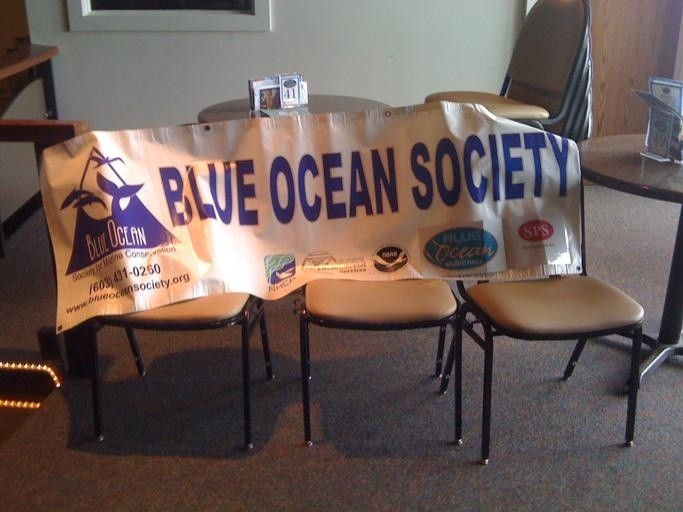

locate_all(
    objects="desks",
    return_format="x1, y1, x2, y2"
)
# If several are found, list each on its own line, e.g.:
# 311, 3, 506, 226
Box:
574, 132, 683, 390
198, 92, 389, 129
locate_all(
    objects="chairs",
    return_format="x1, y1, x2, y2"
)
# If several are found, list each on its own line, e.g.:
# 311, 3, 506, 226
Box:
423, 2, 598, 264
439, 270, 643, 466
87, 294, 276, 451
295, 281, 462, 449
